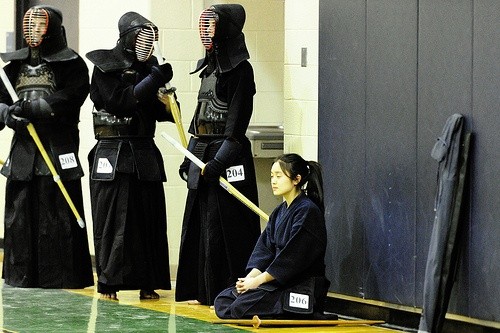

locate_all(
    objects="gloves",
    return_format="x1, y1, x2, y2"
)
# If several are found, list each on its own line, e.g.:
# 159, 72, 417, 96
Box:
180, 161, 190, 181
6, 99, 41, 130
151, 64, 173, 83
202, 160, 223, 181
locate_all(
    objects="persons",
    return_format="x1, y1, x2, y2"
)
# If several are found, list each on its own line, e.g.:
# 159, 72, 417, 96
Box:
85, 12, 182, 300
176, 4, 261, 310
214, 153, 339, 327
0, 5, 94, 289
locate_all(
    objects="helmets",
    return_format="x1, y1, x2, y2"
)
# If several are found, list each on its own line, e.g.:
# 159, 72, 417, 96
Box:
22, 4, 63, 48
119, 12, 158, 63
200, 4, 245, 51
